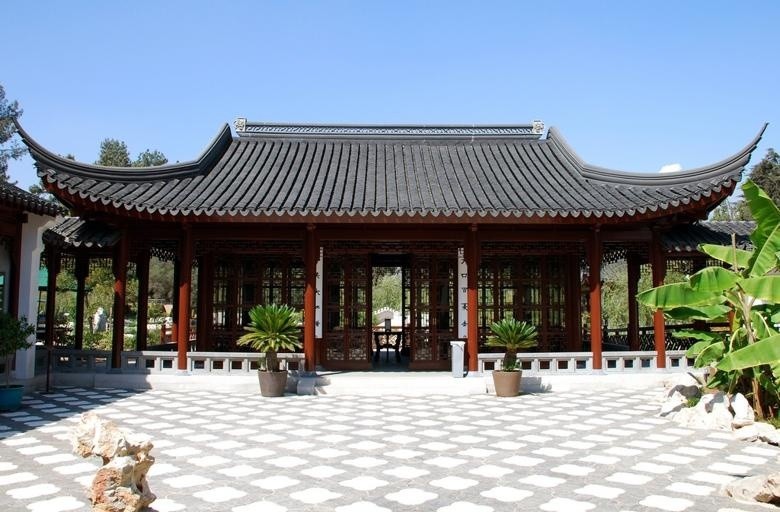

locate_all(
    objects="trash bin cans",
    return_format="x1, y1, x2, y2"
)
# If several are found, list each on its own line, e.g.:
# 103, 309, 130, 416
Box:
450, 340, 466, 378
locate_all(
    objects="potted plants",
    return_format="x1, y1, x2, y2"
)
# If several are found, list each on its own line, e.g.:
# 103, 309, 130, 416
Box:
0, 307, 35, 411
236, 303, 303, 396
484, 318, 539, 396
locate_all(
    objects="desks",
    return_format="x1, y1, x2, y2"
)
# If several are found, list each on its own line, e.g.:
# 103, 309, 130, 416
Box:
373, 332, 402, 360
